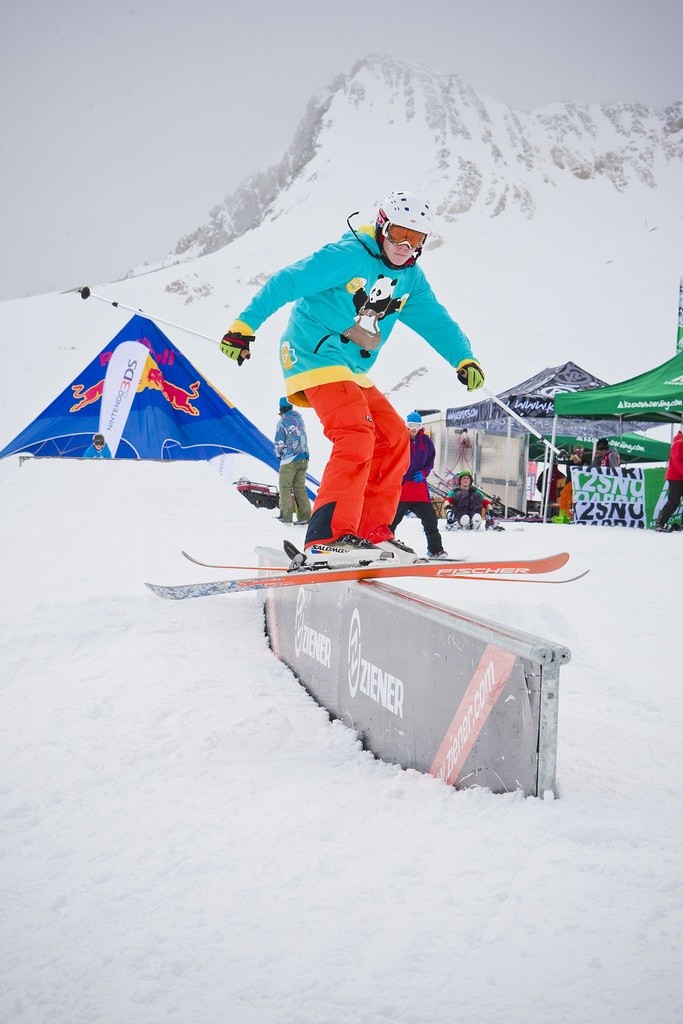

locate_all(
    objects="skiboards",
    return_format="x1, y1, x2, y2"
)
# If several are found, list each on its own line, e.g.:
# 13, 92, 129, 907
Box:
420, 557, 464, 562
147, 548, 592, 601
427, 468, 518, 518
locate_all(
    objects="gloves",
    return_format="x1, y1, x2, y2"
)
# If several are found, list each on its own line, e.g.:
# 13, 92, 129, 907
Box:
412, 468, 427, 483
219, 331, 249, 366
458, 364, 484, 391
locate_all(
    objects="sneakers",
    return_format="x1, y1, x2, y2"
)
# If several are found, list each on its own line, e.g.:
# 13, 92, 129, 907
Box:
372, 538, 417, 565
428, 551, 446, 558
305, 534, 399, 567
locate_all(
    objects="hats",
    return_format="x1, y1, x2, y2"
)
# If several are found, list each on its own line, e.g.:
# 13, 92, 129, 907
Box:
596, 438, 608, 446
406, 412, 422, 422
93, 435, 103, 441
279, 397, 291, 407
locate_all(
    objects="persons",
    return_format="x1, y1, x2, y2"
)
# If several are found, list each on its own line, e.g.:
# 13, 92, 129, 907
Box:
566, 444, 590, 485
588, 438, 621, 466
220, 192, 485, 571
536, 460, 565, 518
387, 411, 448, 558
444, 470, 494, 531
655, 422, 683, 533
274, 396, 311, 525
82, 433, 111, 458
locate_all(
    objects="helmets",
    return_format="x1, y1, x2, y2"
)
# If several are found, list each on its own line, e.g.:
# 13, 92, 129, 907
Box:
458, 471, 473, 486
377, 190, 433, 253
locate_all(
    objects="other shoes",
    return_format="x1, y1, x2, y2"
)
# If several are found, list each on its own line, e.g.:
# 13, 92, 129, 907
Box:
656, 525, 673, 532
471, 513, 481, 530
460, 515, 469, 530
293, 520, 309, 525
276, 517, 291, 524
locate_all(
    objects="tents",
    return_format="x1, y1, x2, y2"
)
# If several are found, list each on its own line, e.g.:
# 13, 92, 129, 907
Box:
445, 361, 669, 522
543, 350, 683, 524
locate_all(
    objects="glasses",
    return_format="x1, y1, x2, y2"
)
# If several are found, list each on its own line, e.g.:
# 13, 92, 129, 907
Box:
381, 221, 425, 249
406, 422, 422, 430
94, 441, 103, 445
